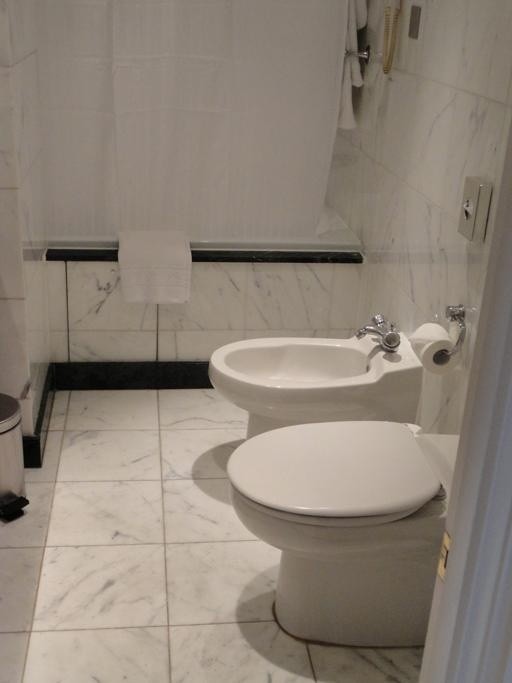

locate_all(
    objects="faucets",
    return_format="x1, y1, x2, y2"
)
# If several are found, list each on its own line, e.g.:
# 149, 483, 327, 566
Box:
355, 313, 401, 353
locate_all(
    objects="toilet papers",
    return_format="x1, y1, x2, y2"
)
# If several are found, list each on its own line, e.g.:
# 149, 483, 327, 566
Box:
407, 322, 461, 375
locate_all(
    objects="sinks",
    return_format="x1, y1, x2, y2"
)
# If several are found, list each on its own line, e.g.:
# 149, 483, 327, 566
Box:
208, 331, 425, 439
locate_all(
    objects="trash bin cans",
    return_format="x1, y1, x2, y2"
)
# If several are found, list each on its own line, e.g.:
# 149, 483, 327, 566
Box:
0, 393, 26, 497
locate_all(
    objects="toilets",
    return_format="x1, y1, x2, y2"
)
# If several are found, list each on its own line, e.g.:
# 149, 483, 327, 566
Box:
226, 421, 448, 648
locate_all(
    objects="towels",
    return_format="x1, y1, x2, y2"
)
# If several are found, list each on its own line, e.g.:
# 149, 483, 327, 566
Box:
116, 228, 192, 306
338, 1, 390, 130
